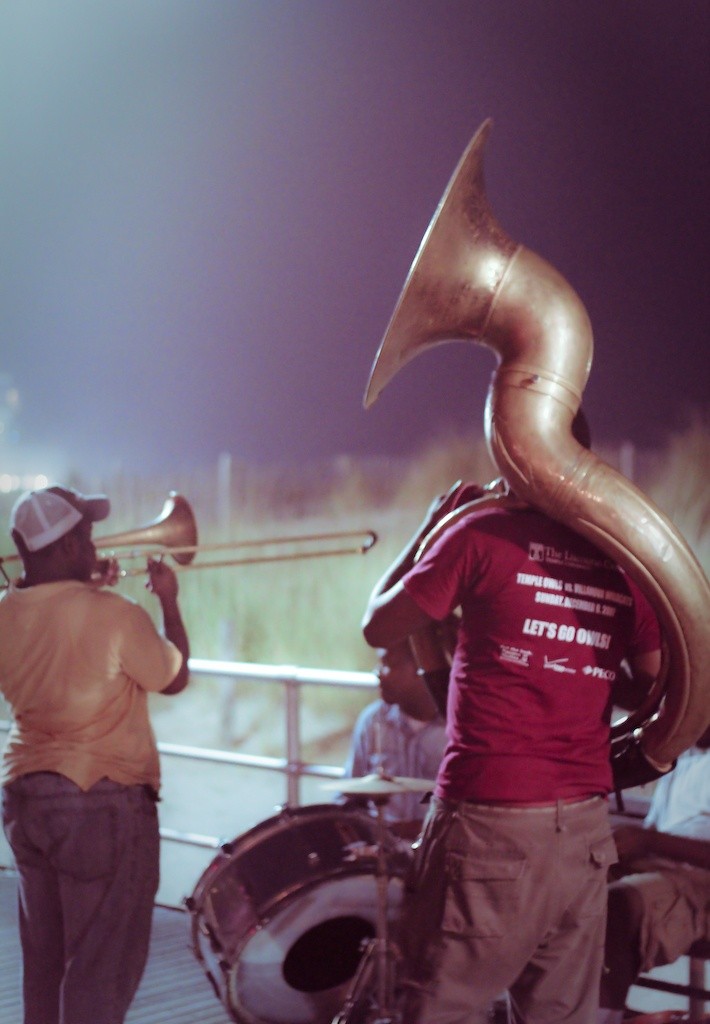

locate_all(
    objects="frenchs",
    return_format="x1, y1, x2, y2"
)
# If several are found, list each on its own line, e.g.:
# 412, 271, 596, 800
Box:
361, 117, 710, 790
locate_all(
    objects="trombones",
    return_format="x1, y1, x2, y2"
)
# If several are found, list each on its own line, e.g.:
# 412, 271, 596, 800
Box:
0, 487, 378, 596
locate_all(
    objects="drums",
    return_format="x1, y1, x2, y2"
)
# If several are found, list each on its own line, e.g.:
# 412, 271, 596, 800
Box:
188, 809, 417, 1024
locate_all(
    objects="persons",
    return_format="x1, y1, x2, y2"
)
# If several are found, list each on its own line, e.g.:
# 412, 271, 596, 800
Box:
0, 487, 190, 1024
599, 729, 710, 1024
339, 646, 447, 840
363, 409, 659, 1024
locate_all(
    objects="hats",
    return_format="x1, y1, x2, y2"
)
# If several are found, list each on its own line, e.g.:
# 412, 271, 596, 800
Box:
11, 486, 110, 554
